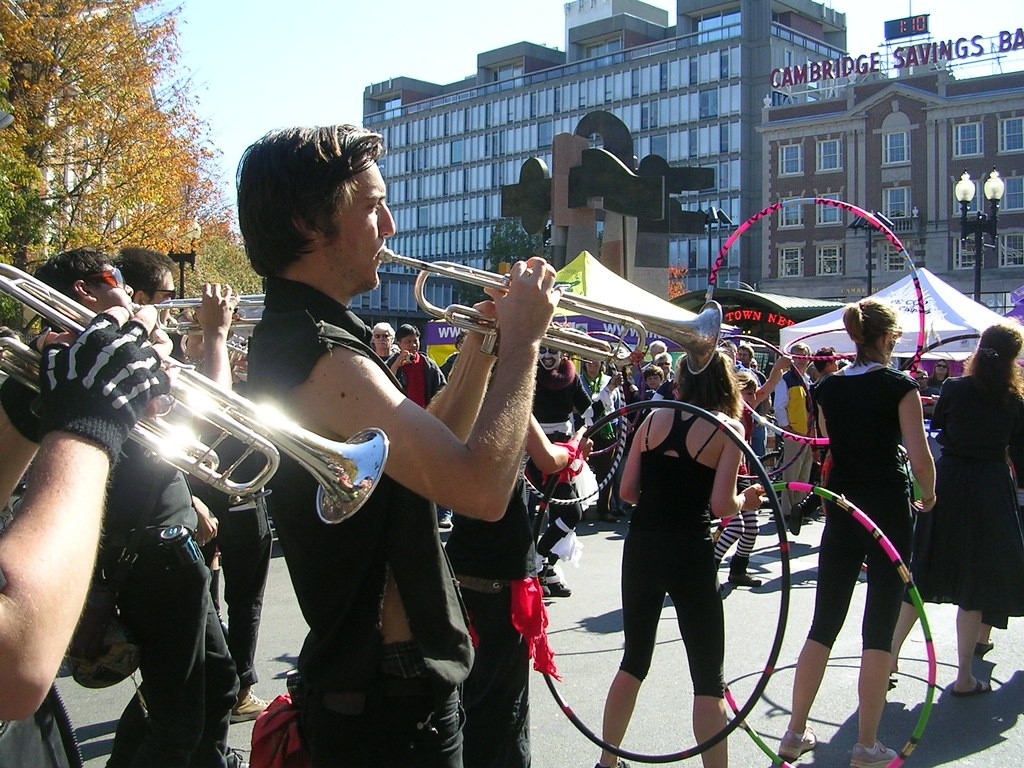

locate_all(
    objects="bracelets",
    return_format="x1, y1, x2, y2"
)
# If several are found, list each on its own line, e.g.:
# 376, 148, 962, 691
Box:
921, 493, 936, 501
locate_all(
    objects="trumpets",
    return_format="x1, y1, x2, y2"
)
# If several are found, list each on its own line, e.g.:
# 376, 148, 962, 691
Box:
179, 332, 249, 383
131, 293, 268, 334
375, 246, 723, 376
0, 264, 391, 526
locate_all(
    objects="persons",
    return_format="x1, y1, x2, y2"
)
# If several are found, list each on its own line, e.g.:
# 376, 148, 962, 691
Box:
777, 301, 937, 768
596, 350, 770, 768
372, 318, 469, 528
239, 125, 562, 768
517, 334, 855, 597
975, 444, 1018, 653
1, 247, 273, 768
444, 409, 594, 767
901, 358, 950, 420
889, 325, 1024, 695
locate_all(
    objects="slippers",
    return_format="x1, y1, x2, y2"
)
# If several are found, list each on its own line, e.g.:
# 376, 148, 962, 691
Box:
951, 678, 991, 697
889, 672, 899, 682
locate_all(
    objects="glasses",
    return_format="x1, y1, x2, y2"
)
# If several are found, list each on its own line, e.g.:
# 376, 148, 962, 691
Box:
374, 333, 391, 339
915, 376, 928, 381
154, 289, 177, 300
936, 364, 947, 368
658, 363, 670, 367
84, 268, 127, 288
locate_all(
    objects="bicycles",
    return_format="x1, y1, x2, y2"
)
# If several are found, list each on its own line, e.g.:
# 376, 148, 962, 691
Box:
751, 442, 823, 505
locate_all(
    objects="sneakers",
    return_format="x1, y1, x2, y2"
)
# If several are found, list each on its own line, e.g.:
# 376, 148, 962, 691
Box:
230, 687, 273, 721
851, 739, 898, 768
778, 729, 817, 758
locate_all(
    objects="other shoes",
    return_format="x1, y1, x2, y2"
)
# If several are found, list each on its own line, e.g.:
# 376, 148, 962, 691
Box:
599, 513, 617, 522
438, 516, 451, 526
610, 506, 627, 516
975, 641, 994, 655
789, 502, 803, 536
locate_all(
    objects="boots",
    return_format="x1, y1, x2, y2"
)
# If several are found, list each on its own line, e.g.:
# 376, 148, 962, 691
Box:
535, 552, 551, 596
548, 552, 571, 597
728, 553, 762, 586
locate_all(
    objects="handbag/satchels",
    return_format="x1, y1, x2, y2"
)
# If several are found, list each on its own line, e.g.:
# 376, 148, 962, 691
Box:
67, 567, 138, 689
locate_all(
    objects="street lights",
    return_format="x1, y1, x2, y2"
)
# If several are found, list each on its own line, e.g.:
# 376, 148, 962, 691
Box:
166, 220, 202, 299
846, 209, 894, 297
955, 164, 1005, 304
